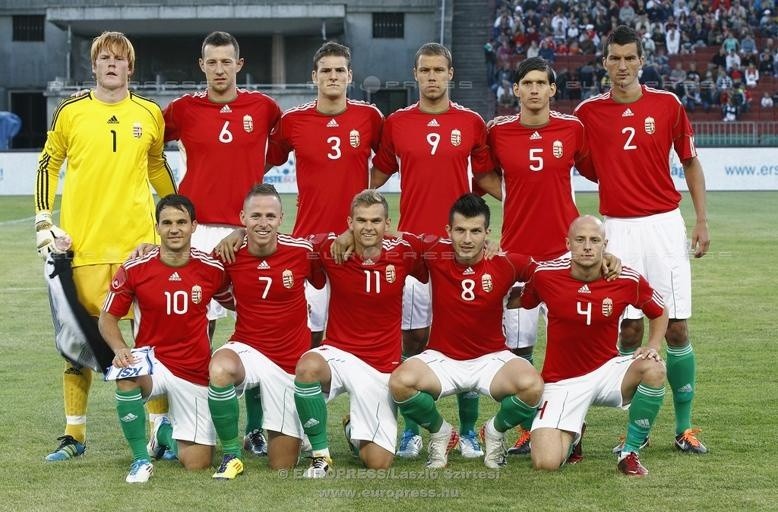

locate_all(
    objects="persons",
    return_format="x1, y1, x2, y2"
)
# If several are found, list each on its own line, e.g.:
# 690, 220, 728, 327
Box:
125, 181, 329, 478
328, 193, 623, 472
264, 41, 384, 348
71, 30, 285, 459
214, 187, 502, 482
507, 213, 669, 475
372, 41, 502, 460
473, 57, 597, 455
485, 0, 777, 122
96, 192, 236, 484
33, 29, 177, 462
571, 25, 713, 454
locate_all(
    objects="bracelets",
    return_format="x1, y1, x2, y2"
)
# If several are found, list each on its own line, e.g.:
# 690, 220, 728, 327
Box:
34, 210, 53, 225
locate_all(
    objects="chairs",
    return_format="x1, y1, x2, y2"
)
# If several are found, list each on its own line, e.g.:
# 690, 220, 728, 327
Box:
495, 0, 778, 135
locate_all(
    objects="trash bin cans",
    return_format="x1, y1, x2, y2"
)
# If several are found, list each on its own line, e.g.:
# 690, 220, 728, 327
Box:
0, 111, 22, 152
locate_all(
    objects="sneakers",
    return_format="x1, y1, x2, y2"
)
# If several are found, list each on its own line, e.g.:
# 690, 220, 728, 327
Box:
125, 458, 154, 483
395, 416, 532, 470
617, 453, 649, 475
342, 414, 359, 459
674, 428, 708, 453
612, 434, 650, 453
566, 420, 586, 465
146, 416, 176, 460
45, 435, 87, 461
302, 456, 332, 479
243, 427, 267, 456
211, 454, 244, 480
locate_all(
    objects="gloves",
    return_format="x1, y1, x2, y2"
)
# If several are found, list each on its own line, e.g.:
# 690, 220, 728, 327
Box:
34, 212, 72, 261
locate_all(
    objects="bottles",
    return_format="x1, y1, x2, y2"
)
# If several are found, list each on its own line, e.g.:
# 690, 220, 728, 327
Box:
55, 236, 73, 251
